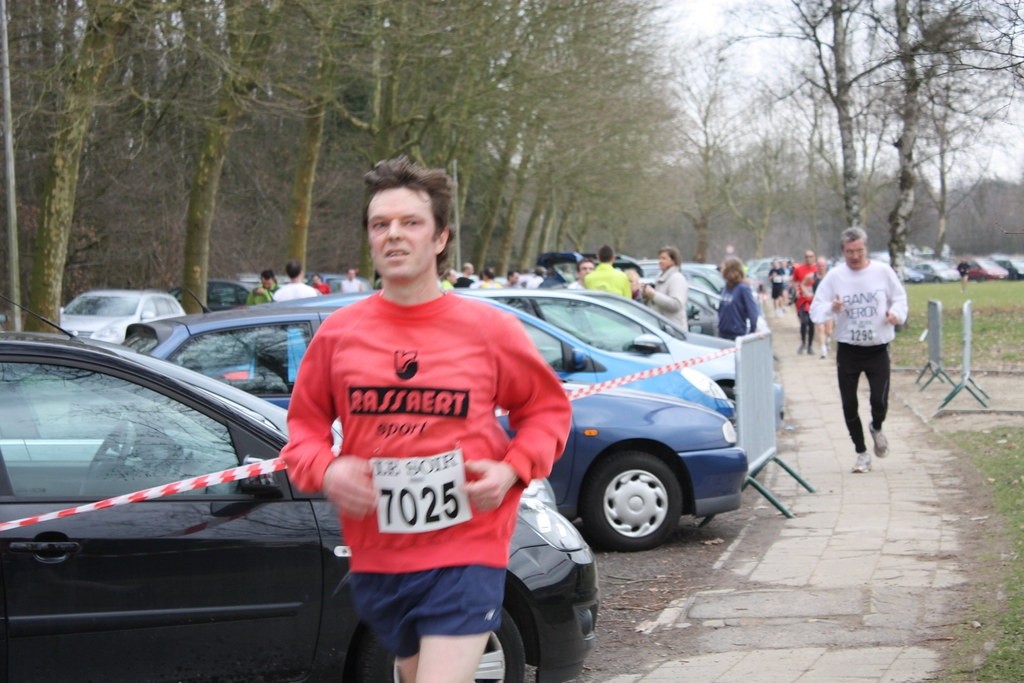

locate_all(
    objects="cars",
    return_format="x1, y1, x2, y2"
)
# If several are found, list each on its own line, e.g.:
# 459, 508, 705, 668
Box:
823, 248, 1024, 282
60, 289, 186, 344
0, 322, 599, 682
122, 306, 749, 550
223, 296, 738, 425
464, 253, 797, 427
169, 273, 377, 309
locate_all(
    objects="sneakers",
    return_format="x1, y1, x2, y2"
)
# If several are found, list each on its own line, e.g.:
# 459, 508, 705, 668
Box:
853, 453, 871, 474
869, 422, 888, 456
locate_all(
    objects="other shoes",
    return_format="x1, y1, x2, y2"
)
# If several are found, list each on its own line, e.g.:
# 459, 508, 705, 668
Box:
807, 345, 813, 354
819, 345, 828, 358
797, 343, 806, 355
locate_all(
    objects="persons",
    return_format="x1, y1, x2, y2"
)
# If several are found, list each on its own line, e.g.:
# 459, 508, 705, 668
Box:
568, 260, 595, 290
311, 273, 331, 296
800, 257, 834, 359
280, 155, 571, 683
624, 268, 649, 306
793, 249, 817, 355
272, 262, 321, 301
441, 262, 546, 290
769, 259, 799, 315
717, 257, 760, 341
642, 246, 689, 332
245, 269, 280, 305
583, 245, 633, 301
809, 227, 910, 474
338, 268, 366, 294
